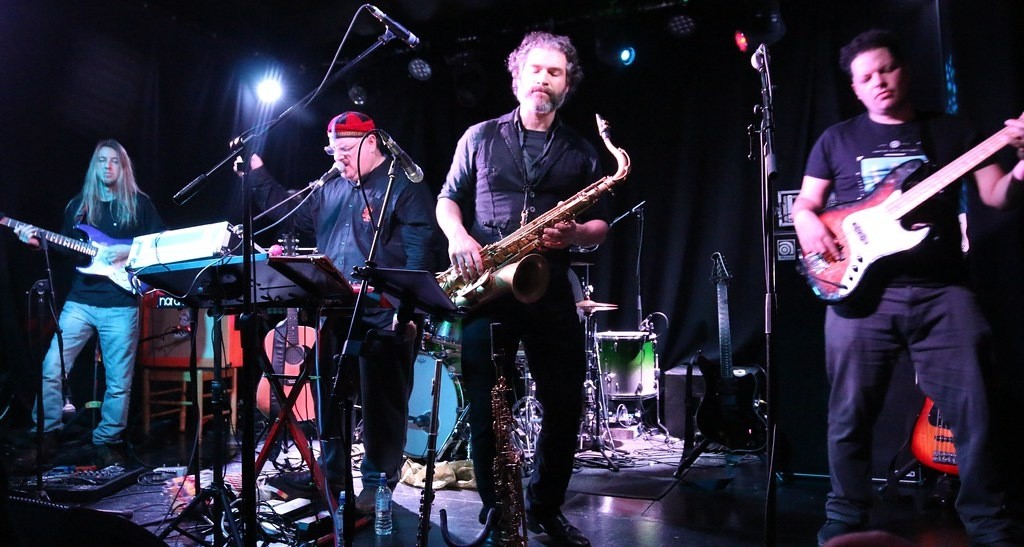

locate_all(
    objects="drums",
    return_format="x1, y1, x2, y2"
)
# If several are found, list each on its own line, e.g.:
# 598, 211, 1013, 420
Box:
426, 311, 465, 351
595, 330, 657, 402
401, 349, 470, 460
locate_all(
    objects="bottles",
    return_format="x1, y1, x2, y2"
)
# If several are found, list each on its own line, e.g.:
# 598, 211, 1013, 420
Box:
374, 472, 393, 536
334, 491, 346, 547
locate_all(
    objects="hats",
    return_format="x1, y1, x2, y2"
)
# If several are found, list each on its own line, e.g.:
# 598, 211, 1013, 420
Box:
327, 110, 377, 139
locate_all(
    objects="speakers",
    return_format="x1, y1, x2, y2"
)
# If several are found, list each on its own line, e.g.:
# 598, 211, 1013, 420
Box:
139, 289, 243, 371
766, 167, 925, 485
0, 495, 170, 547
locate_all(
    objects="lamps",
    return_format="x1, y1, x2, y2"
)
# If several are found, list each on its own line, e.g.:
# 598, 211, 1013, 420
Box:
735, 12, 786, 53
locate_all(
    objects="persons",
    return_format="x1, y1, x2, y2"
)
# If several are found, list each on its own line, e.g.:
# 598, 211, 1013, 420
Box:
436, 32, 614, 547
230, 112, 440, 517
791, 31, 1024, 547
14, 139, 167, 466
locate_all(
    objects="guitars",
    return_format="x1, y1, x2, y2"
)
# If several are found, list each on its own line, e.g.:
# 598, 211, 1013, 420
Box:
910, 395, 959, 473
696, 252, 769, 452
804, 120, 1021, 305
257, 306, 320, 425
0, 215, 156, 296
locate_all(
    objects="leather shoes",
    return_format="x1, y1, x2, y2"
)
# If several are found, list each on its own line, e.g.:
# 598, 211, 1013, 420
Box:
483, 526, 499, 547
524, 509, 591, 547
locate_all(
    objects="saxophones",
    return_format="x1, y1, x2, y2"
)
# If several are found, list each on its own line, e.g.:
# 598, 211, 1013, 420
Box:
435, 112, 633, 321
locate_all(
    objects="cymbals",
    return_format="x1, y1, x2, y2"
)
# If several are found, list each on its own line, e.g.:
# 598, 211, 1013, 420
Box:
573, 300, 619, 315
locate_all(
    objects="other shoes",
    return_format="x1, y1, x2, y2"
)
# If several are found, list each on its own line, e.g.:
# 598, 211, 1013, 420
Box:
355, 487, 377, 513
15, 434, 58, 464
283, 471, 346, 494
816, 519, 853, 546
97, 444, 124, 466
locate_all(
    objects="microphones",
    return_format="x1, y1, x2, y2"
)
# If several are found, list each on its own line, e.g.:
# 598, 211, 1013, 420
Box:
751, 43, 772, 69
314, 161, 346, 189
60, 374, 76, 416
366, 4, 419, 48
639, 314, 653, 331
413, 414, 430, 427
379, 129, 424, 183
172, 324, 192, 333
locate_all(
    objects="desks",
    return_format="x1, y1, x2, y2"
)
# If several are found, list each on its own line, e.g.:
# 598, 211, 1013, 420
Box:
142, 369, 238, 434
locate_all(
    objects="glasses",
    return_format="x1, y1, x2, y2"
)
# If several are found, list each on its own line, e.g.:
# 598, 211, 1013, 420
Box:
324, 137, 363, 155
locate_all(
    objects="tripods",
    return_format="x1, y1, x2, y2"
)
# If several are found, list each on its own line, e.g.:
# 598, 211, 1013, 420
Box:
574, 324, 631, 472
157, 289, 247, 547
510, 372, 544, 453
